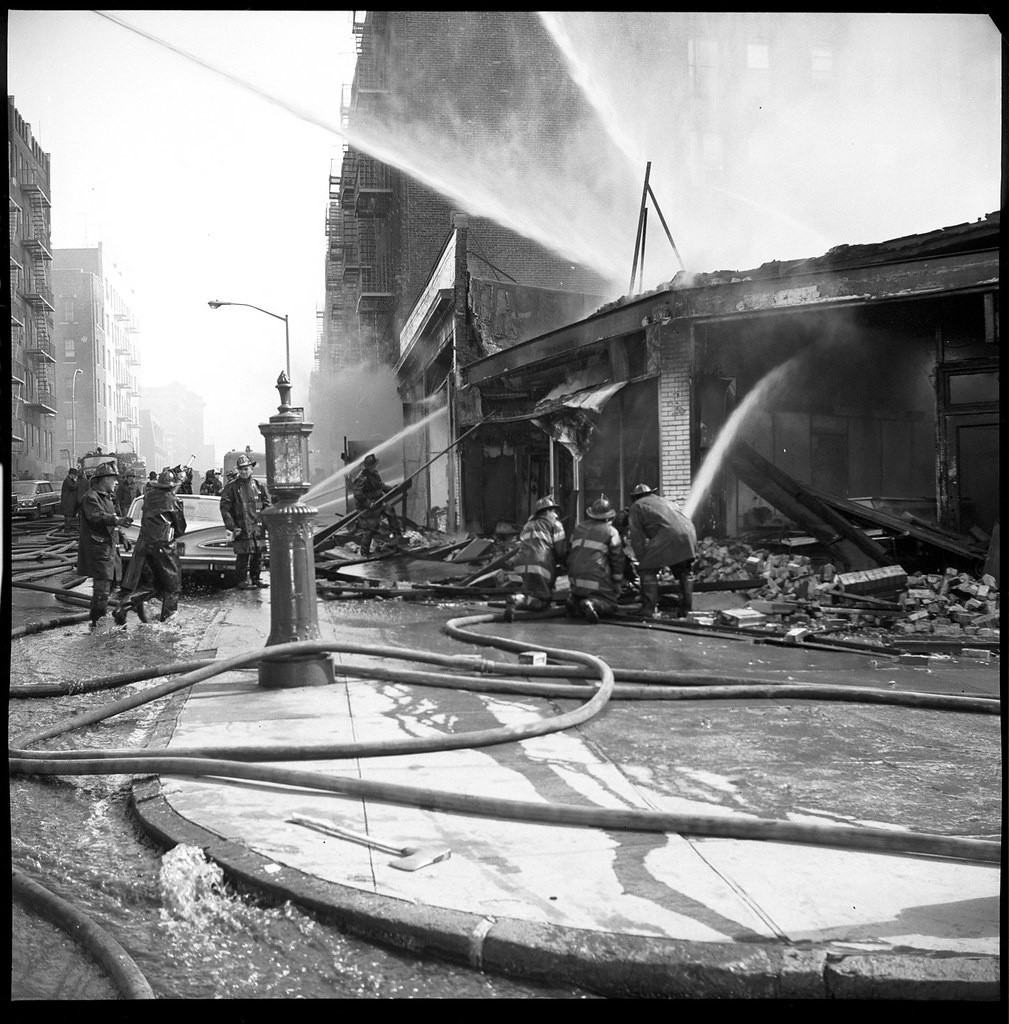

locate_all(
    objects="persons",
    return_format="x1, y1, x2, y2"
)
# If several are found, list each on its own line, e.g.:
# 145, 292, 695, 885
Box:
629, 483, 699, 619
61, 454, 625, 628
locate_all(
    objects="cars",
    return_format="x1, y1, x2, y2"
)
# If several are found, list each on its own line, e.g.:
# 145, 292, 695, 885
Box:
114, 494, 244, 587
12, 480, 61, 521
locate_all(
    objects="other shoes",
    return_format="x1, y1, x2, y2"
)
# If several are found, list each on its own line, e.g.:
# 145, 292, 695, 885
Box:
236, 580, 257, 590
251, 578, 269, 587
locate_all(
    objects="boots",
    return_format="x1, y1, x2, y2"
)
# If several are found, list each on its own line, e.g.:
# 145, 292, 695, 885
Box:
676, 579, 694, 618
571, 598, 611, 623
113, 589, 146, 624
360, 541, 374, 557
504, 593, 542, 623
160, 593, 179, 622
627, 584, 658, 618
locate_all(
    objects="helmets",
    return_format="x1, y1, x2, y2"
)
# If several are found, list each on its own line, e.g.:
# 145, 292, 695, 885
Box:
234, 455, 256, 469
90, 462, 119, 480
149, 469, 182, 488
629, 483, 657, 496
148, 471, 158, 479
586, 498, 616, 519
534, 497, 559, 515
205, 469, 238, 481
126, 471, 136, 477
66, 468, 78, 476
359, 454, 379, 469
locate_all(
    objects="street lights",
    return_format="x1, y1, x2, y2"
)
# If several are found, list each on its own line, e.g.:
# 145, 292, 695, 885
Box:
72, 369, 84, 468
208, 299, 290, 404
